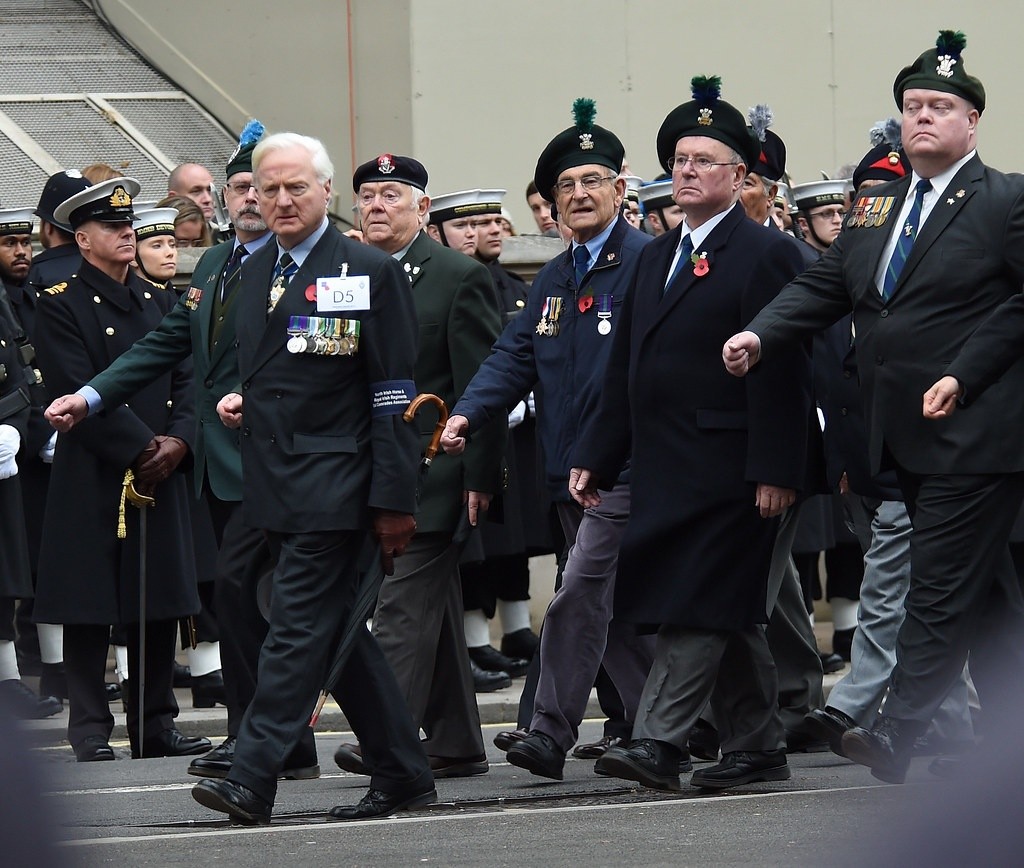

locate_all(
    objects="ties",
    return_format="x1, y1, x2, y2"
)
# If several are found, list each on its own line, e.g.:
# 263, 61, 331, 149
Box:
221, 244, 248, 305
265, 252, 298, 315
573, 244, 591, 288
881, 178, 934, 303
662, 233, 694, 295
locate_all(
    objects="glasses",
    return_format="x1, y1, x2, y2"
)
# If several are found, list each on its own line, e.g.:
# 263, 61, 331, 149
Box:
552, 174, 617, 196
810, 209, 846, 219
667, 155, 739, 173
175, 238, 205, 248
226, 181, 257, 195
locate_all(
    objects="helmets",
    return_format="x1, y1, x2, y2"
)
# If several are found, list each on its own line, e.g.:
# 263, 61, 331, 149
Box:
34, 169, 94, 234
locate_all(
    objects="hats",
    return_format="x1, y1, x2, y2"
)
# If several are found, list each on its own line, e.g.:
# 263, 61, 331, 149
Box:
352, 29, 986, 223
0, 176, 182, 239
225, 119, 267, 180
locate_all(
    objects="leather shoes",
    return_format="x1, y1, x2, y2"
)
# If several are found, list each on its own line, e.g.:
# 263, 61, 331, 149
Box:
190, 668, 225, 709
468, 645, 529, 677
501, 628, 540, 662
76, 735, 116, 762
172, 659, 192, 688
39, 665, 124, 703
186, 707, 1011, 827
832, 625, 857, 663
818, 650, 845, 675
469, 654, 512, 692
131, 727, 213, 759
0, 679, 63, 720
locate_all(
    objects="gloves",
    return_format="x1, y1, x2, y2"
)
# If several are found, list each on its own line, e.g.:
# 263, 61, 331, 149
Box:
527, 391, 536, 418
507, 400, 526, 429
0, 424, 21, 480
131, 434, 189, 489
371, 507, 419, 576
38, 430, 59, 465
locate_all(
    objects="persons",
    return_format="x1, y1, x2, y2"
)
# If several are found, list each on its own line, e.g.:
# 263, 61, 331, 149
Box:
0, 30, 1024, 829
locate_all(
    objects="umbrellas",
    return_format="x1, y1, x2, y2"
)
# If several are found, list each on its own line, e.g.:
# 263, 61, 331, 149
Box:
309, 395, 447, 726
